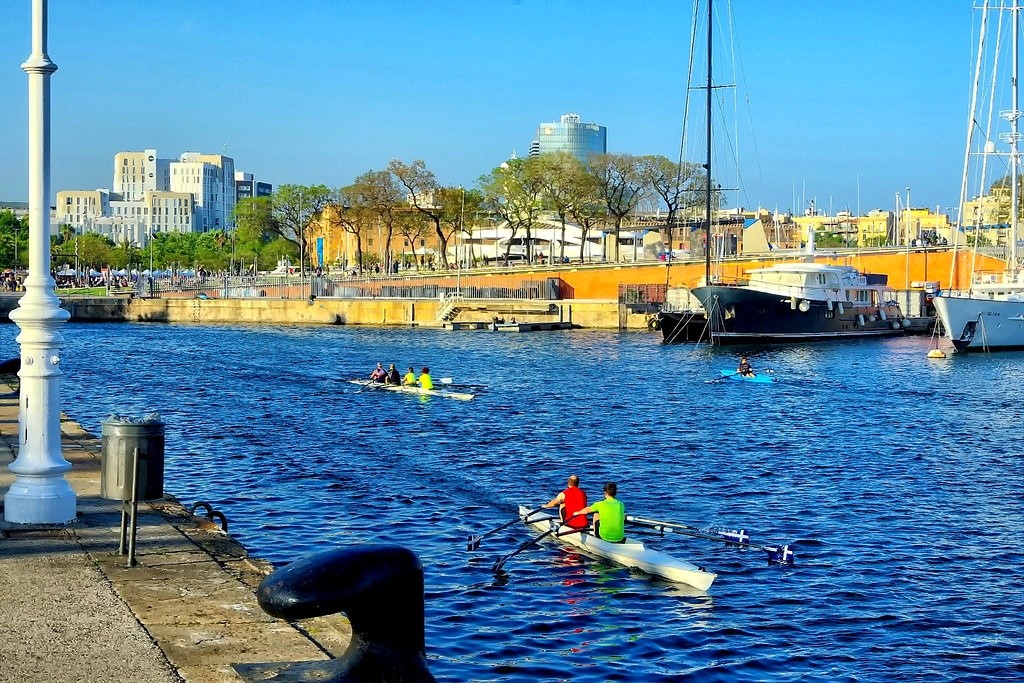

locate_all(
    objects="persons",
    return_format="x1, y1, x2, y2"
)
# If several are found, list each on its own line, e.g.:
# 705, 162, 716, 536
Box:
0, 269, 255, 288
384, 363, 400, 386
290, 254, 490, 280
660, 253, 667, 261
736, 358, 755, 378
537, 251, 569, 263
416, 367, 434, 390
401, 366, 417, 388
541, 475, 589, 532
923, 232, 937, 247
573, 482, 627, 544
371, 362, 388, 384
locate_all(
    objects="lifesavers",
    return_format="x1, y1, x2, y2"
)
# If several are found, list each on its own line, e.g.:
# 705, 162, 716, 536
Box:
648, 318, 660, 330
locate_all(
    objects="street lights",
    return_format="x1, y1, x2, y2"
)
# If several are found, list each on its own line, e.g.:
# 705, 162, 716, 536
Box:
457, 190, 466, 298
298, 193, 303, 300
150, 194, 158, 277
15, 228, 21, 281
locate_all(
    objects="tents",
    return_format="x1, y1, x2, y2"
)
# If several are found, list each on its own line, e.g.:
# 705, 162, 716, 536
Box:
402, 247, 437, 254
57, 268, 190, 277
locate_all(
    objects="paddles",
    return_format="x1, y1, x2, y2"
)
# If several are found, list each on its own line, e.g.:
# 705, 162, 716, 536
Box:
626, 515, 750, 543
467, 509, 542, 551
358, 380, 374, 390
432, 384, 489, 388
626, 520, 794, 562
752, 369, 774, 373
711, 371, 743, 380
492, 515, 577, 571
355, 384, 386, 393
401, 377, 452, 383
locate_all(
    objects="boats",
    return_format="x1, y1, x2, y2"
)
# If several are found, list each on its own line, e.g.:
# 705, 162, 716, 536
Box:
345, 377, 475, 402
518, 505, 718, 592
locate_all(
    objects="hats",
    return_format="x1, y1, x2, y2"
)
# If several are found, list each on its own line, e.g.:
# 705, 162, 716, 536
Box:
390, 364, 395, 369
377, 362, 383, 367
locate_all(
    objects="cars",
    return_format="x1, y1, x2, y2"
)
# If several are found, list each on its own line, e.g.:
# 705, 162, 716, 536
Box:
269, 262, 383, 281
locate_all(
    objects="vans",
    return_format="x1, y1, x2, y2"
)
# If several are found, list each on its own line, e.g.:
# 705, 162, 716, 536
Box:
444, 292, 464, 298
500, 253, 527, 267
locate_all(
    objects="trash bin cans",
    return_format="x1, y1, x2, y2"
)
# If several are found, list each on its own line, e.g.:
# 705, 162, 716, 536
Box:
99, 420, 165, 502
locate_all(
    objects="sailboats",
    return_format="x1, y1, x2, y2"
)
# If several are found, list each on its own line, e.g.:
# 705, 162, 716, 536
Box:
925, 0, 1023, 356
644, 0, 911, 350
